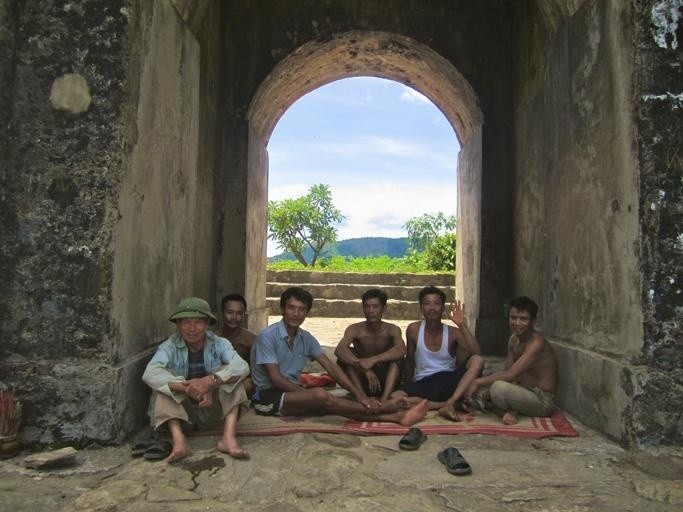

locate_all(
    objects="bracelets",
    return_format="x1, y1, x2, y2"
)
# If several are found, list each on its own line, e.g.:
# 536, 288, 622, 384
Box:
209, 373, 222, 386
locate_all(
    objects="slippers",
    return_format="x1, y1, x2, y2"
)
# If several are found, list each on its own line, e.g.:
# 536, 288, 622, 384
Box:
437, 448, 472, 476
131, 430, 161, 456
399, 427, 426, 450
144, 440, 173, 460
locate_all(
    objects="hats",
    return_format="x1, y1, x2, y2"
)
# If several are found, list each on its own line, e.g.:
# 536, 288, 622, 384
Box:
168, 297, 217, 326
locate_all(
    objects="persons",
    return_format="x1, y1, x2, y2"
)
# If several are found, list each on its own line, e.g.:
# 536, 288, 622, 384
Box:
335, 290, 406, 403
390, 286, 486, 422
216, 292, 258, 400
465, 297, 559, 425
142, 296, 254, 463
250, 288, 428, 427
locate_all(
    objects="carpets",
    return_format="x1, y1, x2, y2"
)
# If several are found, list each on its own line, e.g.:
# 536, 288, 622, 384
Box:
164, 370, 579, 440
